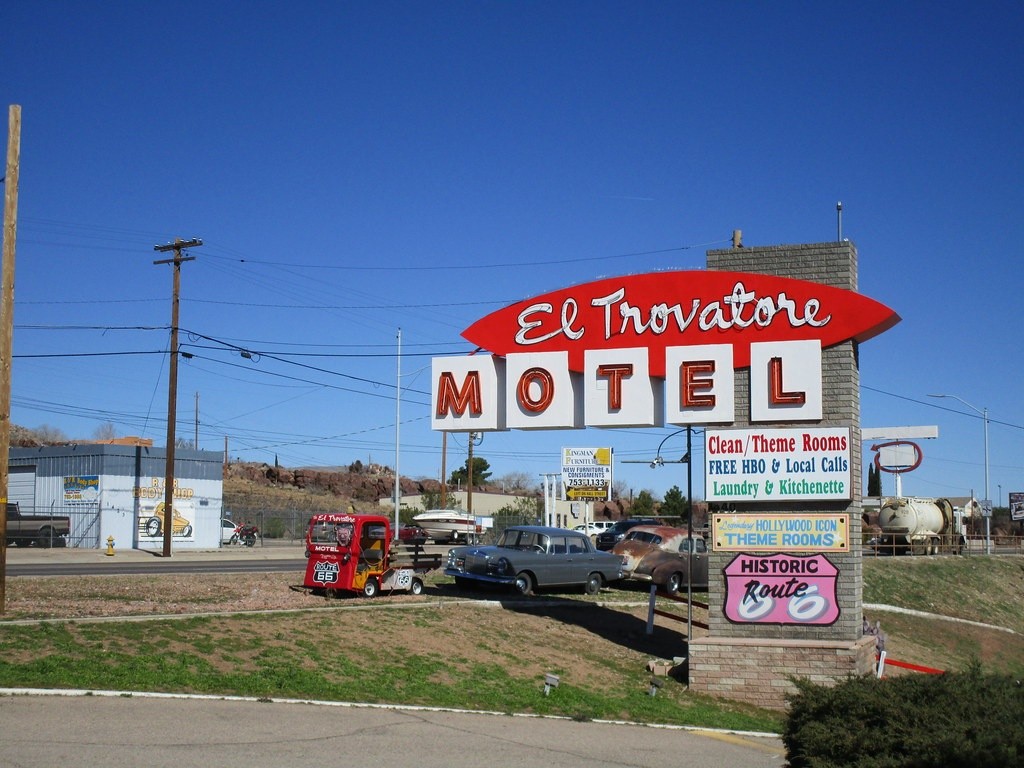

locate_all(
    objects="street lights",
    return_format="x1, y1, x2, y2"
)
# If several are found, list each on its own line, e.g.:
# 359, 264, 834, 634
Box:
925, 393, 991, 555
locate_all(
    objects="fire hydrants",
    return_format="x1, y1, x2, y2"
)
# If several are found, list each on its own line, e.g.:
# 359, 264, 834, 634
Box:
105, 535, 116, 556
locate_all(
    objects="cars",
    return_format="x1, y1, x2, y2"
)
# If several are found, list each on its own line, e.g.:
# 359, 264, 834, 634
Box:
442, 525, 630, 599
570, 517, 709, 597
368, 522, 429, 545
221, 518, 243, 544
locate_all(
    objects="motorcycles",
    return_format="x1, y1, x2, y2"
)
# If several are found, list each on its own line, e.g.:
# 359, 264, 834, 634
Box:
229, 522, 259, 547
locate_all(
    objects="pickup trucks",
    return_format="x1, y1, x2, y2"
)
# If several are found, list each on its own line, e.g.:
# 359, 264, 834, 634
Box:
7, 502, 70, 547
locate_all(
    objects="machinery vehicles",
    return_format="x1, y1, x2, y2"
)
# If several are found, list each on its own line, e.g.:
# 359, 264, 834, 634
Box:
876, 495, 968, 557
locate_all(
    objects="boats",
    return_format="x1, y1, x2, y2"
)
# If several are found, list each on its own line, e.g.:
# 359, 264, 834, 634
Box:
411, 509, 494, 540
